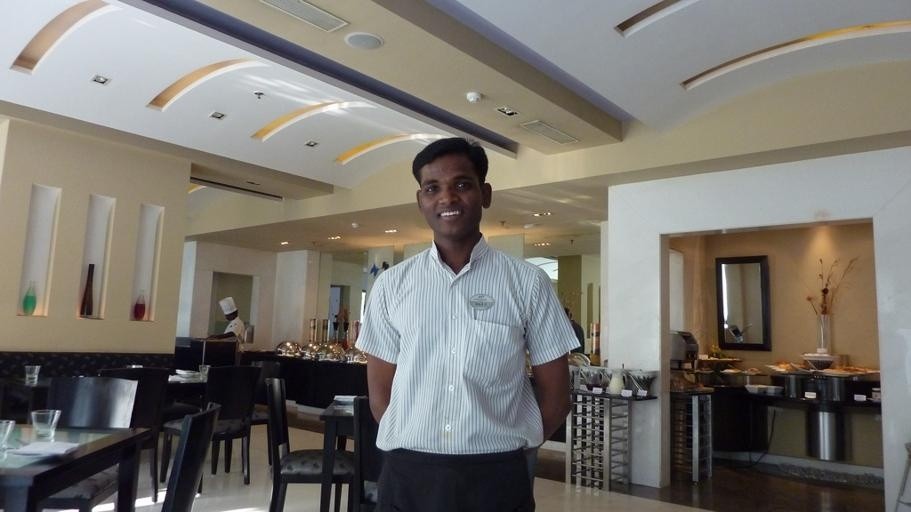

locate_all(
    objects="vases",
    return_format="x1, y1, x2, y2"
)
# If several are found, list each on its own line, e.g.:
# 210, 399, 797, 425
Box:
815, 313, 836, 356
22, 280, 37, 315
81, 263, 94, 317
133, 288, 146, 321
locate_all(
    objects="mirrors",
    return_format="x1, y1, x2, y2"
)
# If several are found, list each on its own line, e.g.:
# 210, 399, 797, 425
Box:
715, 254, 771, 352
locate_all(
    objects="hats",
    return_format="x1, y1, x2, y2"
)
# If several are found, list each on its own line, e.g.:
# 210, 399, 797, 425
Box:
218, 297, 238, 316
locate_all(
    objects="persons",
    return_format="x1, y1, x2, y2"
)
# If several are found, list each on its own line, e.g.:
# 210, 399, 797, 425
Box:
207, 296, 246, 344
563, 308, 584, 354
353, 136, 582, 512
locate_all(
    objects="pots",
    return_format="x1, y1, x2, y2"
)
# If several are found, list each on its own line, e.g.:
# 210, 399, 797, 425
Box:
696, 353, 882, 402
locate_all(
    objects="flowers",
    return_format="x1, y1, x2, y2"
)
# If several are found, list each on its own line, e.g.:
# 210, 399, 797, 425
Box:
806, 257, 855, 315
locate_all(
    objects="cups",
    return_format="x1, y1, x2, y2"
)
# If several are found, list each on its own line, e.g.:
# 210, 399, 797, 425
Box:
579, 365, 656, 396
0, 364, 62, 450
199, 365, 212, 381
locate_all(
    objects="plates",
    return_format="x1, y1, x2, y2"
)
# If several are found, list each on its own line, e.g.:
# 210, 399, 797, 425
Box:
174, 368, 200, 379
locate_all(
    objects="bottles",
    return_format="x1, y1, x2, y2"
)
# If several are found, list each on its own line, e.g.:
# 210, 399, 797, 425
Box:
725, 318, 744, 343
